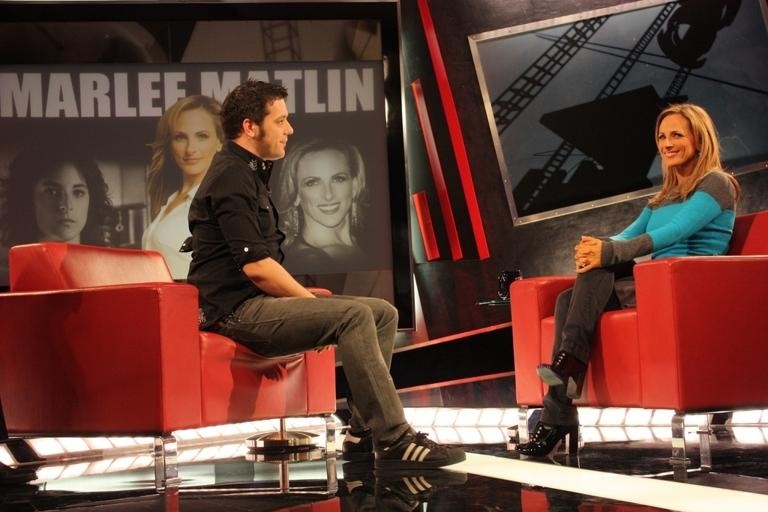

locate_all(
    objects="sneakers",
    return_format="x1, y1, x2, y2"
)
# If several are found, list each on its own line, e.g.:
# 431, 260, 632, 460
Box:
343, 430, 374, 461
373, 470, 467, 508
374, 428, 465, 470
343, 462, 375, 494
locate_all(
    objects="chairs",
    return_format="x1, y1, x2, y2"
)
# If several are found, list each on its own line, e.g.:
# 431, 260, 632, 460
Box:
509, 211, 768, 482
0, 243, 340, 494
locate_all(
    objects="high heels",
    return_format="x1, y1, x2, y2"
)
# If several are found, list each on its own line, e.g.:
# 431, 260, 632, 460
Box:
516, 420, 578, 455
538, 350, 588, 399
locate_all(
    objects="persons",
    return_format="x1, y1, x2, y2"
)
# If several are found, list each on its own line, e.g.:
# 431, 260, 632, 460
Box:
515, 103, 741, 458
137, 94, 226, 283
179, 81, 466, 470
276, 134, 373, 271
334, 460, 468, 512
0, 135, 121, 291
